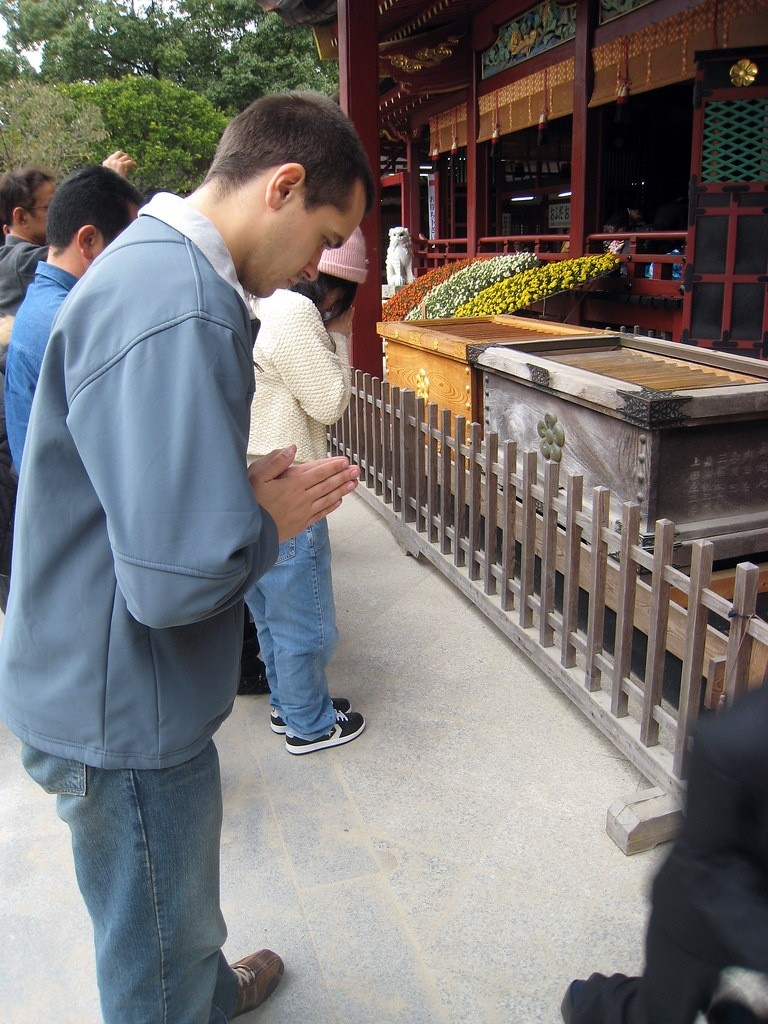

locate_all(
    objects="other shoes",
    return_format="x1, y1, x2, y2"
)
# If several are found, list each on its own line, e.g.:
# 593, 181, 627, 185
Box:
238, 673, 272, 695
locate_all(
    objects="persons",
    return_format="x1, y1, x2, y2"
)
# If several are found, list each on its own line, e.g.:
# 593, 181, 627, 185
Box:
559, 682, 767, 1024
593, 201, 684, 280
0, 92, 369, 1024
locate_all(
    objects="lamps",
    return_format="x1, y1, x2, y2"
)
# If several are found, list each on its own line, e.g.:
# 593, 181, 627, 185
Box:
557, 191, 572, 197
510, 194, 536, 202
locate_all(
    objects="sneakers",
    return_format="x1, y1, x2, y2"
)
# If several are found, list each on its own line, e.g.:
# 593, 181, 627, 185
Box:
228, 948, 285, 1020
285, 709, 366, 755
270, 698, 352, 734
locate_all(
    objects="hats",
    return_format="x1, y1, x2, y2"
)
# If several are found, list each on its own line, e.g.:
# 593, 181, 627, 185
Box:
317, 225, 368, 283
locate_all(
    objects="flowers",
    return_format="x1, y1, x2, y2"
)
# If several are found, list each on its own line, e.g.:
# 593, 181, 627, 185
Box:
403, 253, 541, 322
454, 253, 620, 312
381, 257, 492, 322
